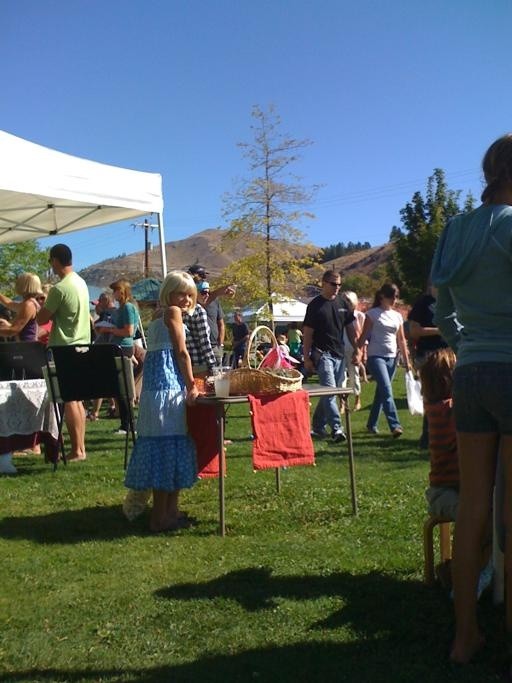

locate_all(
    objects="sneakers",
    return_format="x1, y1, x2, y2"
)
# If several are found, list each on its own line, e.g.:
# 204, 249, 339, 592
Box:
392, 426, 403, 438
311, 428, 329, 438
367, 425, 379, 435
331, 429, 345, 443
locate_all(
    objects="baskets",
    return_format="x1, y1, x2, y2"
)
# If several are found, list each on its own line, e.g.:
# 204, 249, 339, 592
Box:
228, 324, 303, 394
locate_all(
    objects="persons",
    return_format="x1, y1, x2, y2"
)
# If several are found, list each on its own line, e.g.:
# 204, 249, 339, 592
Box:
408, 284, 449, 449
436, 134, 510, 664
420, 348, 457, 521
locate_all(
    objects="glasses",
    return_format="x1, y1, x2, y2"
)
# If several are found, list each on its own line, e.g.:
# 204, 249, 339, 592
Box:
200, 290, 209, 295
329, 281, 341, 286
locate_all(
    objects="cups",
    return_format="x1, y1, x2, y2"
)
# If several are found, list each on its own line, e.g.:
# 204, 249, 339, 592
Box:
211, 365, 234, 397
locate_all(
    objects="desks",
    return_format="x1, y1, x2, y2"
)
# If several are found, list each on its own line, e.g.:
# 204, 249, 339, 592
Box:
197, 382, 357, 540
0, 377, 66, 475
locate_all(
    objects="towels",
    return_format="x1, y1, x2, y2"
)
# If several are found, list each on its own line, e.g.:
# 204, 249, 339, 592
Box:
185, 402, 231, 480
249, 388, 319, 474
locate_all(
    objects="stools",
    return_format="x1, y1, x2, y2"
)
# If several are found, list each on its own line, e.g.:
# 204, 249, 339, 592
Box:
422, 516, 456, 587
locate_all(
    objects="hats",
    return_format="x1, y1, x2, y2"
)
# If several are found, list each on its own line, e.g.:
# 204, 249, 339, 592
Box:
196, 281, 208, 289
189, 265, 210, 275
480, 132, 512, 202
